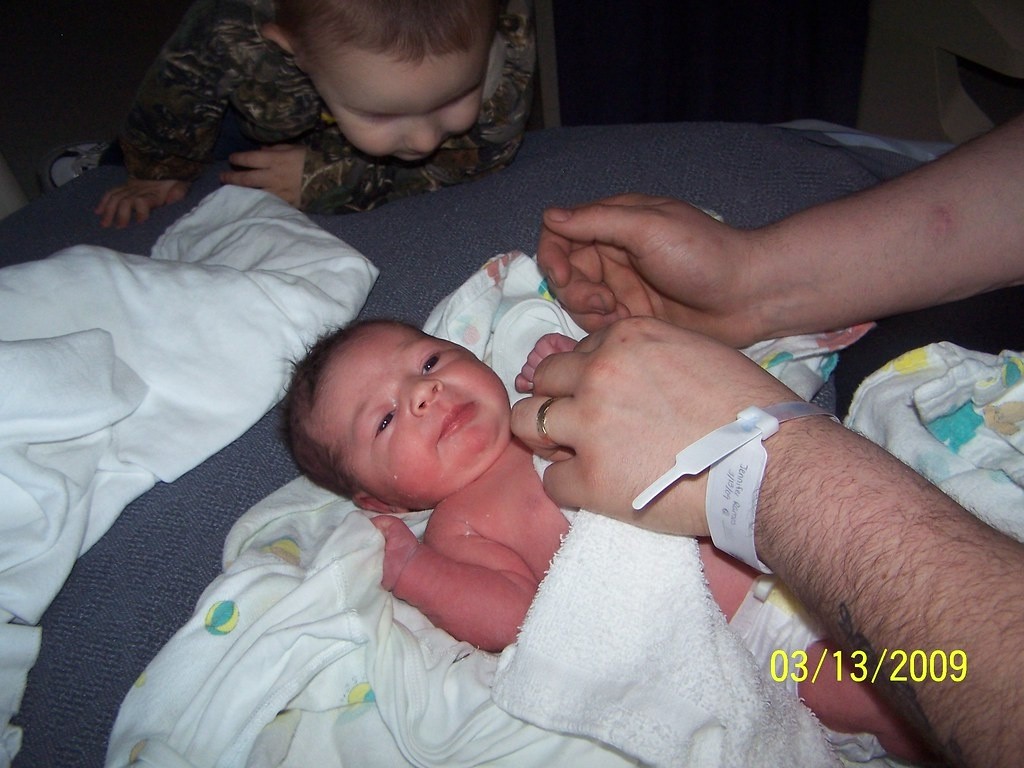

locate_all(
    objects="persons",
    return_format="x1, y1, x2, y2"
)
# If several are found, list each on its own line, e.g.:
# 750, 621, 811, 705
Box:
92, 0, 539, 235
281, 317, 937, 767
506, 106, 1024, 767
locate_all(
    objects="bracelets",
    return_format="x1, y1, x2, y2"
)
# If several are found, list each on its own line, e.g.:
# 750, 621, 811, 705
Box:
633, 401, 841, 575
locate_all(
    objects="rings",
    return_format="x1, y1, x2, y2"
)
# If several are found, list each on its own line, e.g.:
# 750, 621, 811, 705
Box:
536, 395, 564, 449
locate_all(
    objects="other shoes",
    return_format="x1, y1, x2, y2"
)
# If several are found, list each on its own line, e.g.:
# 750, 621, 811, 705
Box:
42, 141, 114, 193
